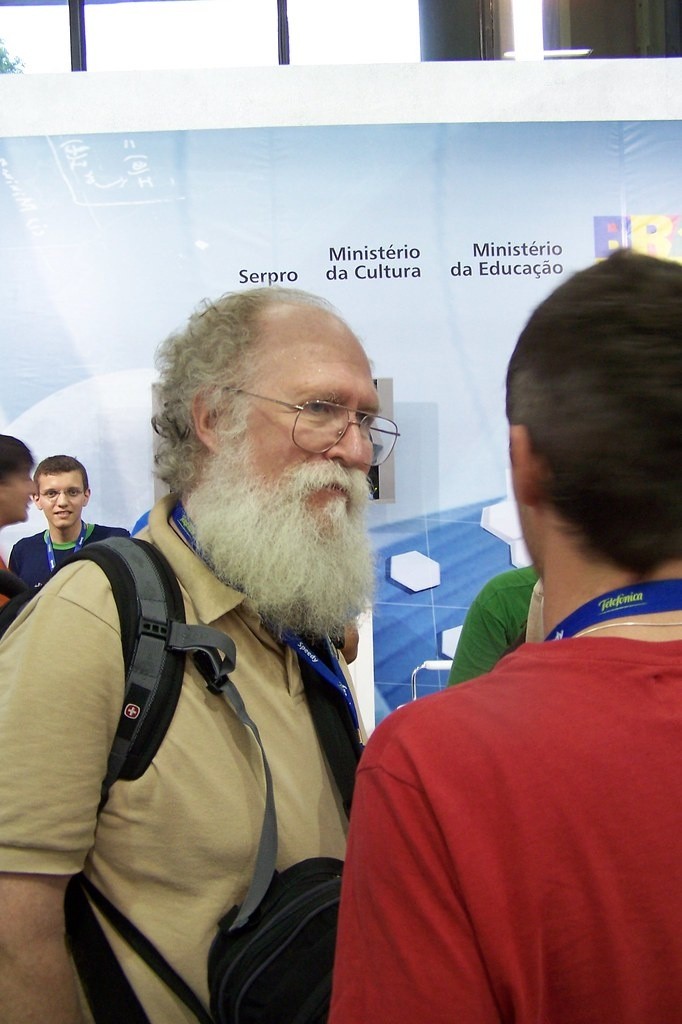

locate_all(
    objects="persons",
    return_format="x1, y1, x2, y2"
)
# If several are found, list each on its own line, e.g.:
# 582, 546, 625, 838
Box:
0, 455, 131, 640
328, 248, 682, 1024
0, 435, 38, 640
446, 565, 540, 690
0, 286, 400, 1024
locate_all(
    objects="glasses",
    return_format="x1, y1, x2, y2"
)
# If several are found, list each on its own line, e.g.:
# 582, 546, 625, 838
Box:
226, 386, 400, 467
36, 488, 85, 499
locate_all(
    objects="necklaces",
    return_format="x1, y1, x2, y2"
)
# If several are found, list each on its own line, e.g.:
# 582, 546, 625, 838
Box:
570, 622, 682, 637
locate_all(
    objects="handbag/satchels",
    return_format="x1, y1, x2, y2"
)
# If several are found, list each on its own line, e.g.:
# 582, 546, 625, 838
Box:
209, 855, 345, 1024
